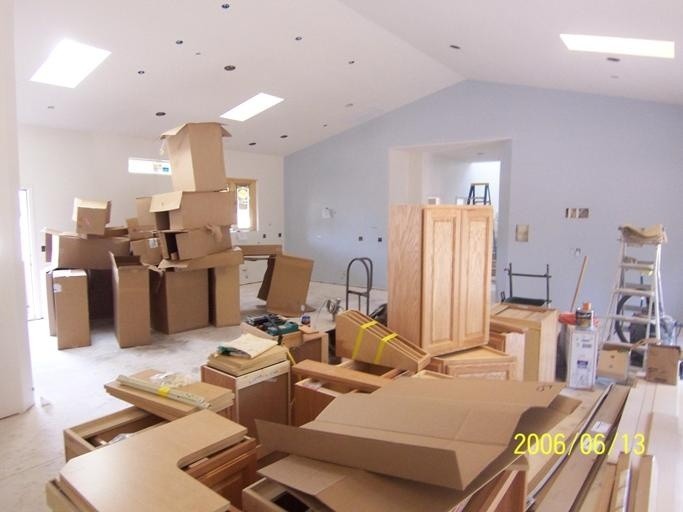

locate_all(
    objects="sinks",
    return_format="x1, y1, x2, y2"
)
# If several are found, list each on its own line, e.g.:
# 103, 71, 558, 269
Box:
301, 310, 335, 333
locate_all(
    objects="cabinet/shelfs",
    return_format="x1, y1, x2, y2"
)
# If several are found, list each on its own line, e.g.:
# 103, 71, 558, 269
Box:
387, 202, 493, 357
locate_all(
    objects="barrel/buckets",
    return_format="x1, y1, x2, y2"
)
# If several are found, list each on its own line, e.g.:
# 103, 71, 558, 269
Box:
576, 302, 594, 328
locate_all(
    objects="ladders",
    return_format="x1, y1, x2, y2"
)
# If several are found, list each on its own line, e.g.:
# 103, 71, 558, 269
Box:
596, 224, 664, 373
466, 182, 497, 283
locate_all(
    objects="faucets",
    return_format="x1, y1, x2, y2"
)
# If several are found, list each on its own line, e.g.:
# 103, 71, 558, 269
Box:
326, 297, 341, 322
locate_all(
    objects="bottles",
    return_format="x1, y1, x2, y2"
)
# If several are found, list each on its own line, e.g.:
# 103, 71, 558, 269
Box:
301, 316, 310, 327
